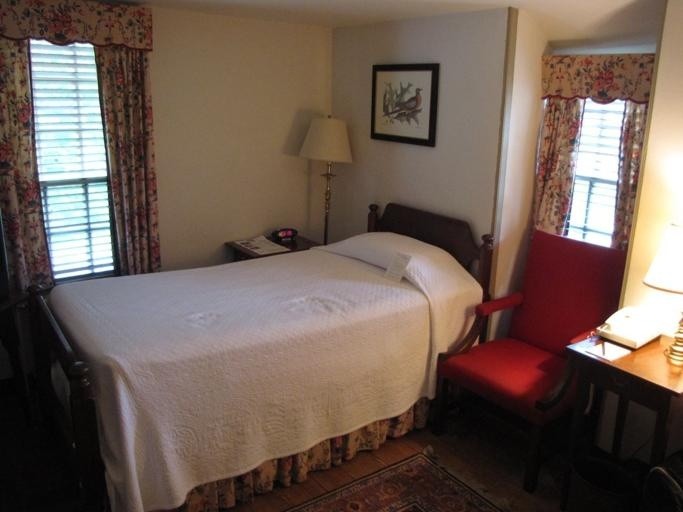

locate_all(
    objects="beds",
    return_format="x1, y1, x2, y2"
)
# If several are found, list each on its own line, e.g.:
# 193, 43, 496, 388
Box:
24, 201, 493, 512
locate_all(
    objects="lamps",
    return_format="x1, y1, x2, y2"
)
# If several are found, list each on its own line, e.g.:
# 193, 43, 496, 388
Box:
296, 114, 354, 245
640, 221, 683, 367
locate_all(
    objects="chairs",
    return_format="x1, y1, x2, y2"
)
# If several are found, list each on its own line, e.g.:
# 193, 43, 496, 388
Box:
427, 227, 629, 495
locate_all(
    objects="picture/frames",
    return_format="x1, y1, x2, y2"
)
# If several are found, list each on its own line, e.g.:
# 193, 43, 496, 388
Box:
369, 62, 440, 149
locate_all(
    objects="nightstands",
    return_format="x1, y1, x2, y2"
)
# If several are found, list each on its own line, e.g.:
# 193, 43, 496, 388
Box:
562, 323, 682, 508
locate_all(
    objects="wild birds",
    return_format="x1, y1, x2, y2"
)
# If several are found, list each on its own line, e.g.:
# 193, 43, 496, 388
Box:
393, 86, 424, 113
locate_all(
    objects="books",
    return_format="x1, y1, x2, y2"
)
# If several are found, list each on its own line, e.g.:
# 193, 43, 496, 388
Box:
234, 232, 292, 256
382, 252, 412, 283
585, 340, 631, 362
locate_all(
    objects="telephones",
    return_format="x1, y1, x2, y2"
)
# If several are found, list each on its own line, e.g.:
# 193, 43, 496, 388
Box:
596, 307, 662, 349
